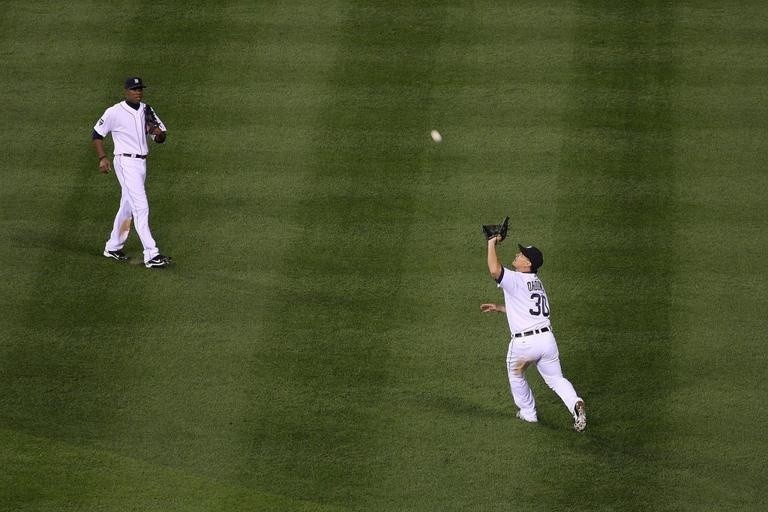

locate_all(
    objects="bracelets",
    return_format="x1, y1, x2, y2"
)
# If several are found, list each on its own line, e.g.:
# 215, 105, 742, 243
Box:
99, 156, 107, 160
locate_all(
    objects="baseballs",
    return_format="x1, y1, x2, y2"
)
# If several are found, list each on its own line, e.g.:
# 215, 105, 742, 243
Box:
431, 130, 441, 143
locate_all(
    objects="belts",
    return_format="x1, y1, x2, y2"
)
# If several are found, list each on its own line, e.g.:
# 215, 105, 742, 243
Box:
124, 154, 147, 159
515, 327, 549, 337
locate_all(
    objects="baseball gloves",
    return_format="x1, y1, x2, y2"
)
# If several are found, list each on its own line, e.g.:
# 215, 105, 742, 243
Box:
145, 104, 164, 141
483, 216, 510, 245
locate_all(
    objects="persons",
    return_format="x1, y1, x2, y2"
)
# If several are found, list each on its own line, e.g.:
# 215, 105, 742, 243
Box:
480, 216, 587, 433
92, 77, 173, 269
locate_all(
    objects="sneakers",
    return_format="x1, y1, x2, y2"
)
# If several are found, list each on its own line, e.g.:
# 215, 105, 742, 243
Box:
573, 401, 587, 433
515, 411, 527, 420
103, 247, 130, 261
145, 255, 173, 268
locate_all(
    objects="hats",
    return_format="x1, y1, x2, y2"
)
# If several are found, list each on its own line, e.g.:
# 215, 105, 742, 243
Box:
126, 77, 146, 90
517, 243, 543, 269
